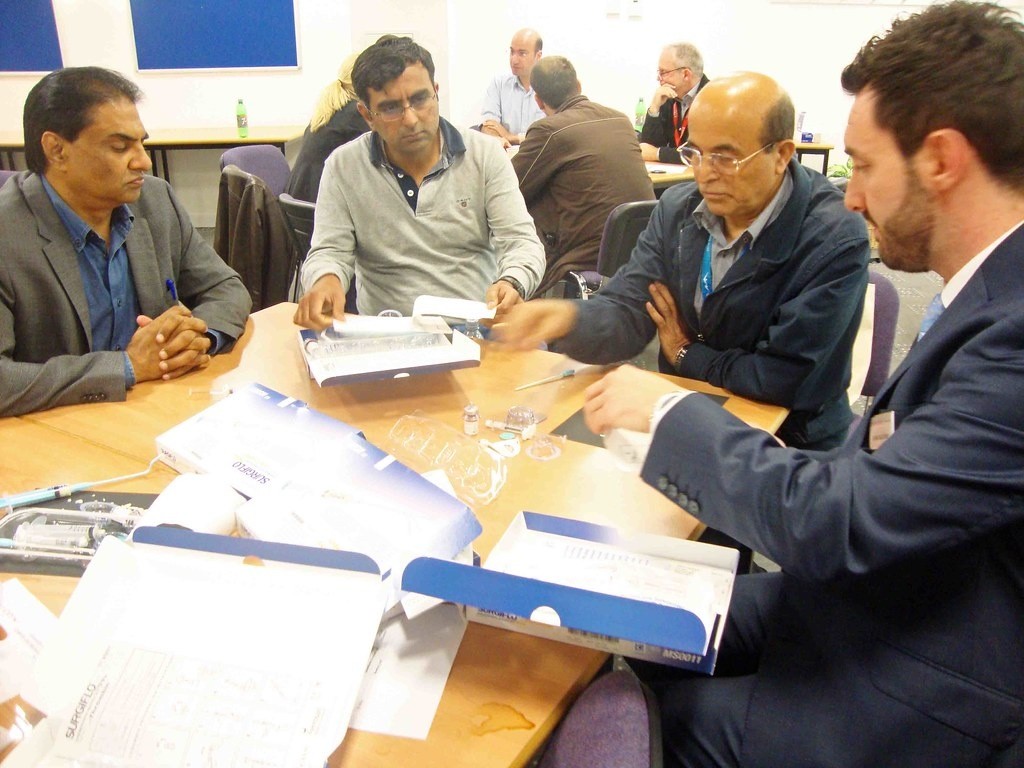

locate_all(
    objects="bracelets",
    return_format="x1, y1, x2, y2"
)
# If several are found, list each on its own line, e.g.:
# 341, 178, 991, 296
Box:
674, 345, 690, 371
495, 277, 525, 298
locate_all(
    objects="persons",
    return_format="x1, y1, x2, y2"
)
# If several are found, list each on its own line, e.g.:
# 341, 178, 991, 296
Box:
586, 0, 1024, 768
512, 57, 656, 297
479, 29, 546, 151
287, 51, 371, 202
0, 66, 252, 417
637, 41, 708, 164
293, 37, 548, 353
499, 70, 871, 573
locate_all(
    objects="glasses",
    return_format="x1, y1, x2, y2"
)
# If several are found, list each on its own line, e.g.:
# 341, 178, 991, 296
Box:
364, 92, 436, 121
676, 142, 773, 175
657, 66, 688, 81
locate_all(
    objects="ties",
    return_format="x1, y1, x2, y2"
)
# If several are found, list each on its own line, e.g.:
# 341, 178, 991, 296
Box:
917, 295, 946, 342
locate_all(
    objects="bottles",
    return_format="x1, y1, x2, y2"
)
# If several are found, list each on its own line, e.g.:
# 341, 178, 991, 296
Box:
236, 99, 247, 137
634, 99, 646, 131
463, 406, 480, 436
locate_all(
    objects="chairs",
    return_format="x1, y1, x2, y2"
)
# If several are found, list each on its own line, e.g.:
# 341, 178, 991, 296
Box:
217, 145, 900, 768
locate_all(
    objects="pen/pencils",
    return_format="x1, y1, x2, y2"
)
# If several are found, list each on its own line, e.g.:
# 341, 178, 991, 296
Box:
165, 279, 176, 300
513, 369, 575, 391
650, 170, 667, 174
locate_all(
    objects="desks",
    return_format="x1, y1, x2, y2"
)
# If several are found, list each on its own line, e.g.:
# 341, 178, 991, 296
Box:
0, 128, 307, 186
0, 299, 793, 767
793, 141, 836, 179
504, 147, 697, 190
